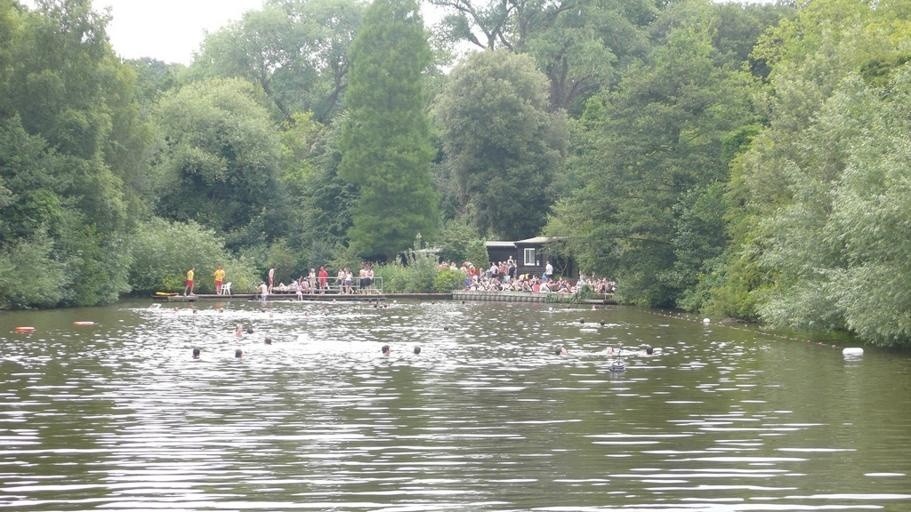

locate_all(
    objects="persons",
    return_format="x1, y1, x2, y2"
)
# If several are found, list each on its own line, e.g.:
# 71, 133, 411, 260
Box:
580, 317, 586, 323
592, 305, 598, 310
184, 267, 197, 296
413, 346, 421, 354
210, 266, 226, 295
437, 255, 616, 294
173, 306, 339, 359
255, 263, 375, 299
461, 300, 466, 305
432, 300, 436, 305
646, 345, 654, 354
555, 345, 568, 356
367, 300, 388, 310
601, 320, 607, 326
444, 326, 449, 331
381, 344, 391, 356
549, 307, 556, 313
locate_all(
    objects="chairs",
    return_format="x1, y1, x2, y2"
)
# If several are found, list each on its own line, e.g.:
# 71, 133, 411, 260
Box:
220, 281, 232, 296
